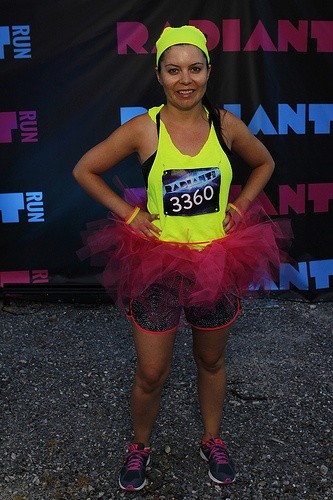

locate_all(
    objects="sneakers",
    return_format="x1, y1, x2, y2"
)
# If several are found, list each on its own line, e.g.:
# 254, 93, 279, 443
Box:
118, 444, 152, 491
200, 434, 237, 485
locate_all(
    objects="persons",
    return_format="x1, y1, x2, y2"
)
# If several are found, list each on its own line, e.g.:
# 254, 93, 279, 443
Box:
71, 24, 292, 492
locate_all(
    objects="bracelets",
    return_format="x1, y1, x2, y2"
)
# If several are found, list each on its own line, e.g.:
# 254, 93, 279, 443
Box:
226, 201, 245, 220
125, 204, 140, 226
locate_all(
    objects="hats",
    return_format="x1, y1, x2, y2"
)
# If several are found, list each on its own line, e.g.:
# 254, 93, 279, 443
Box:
155, 25, 211, 67
